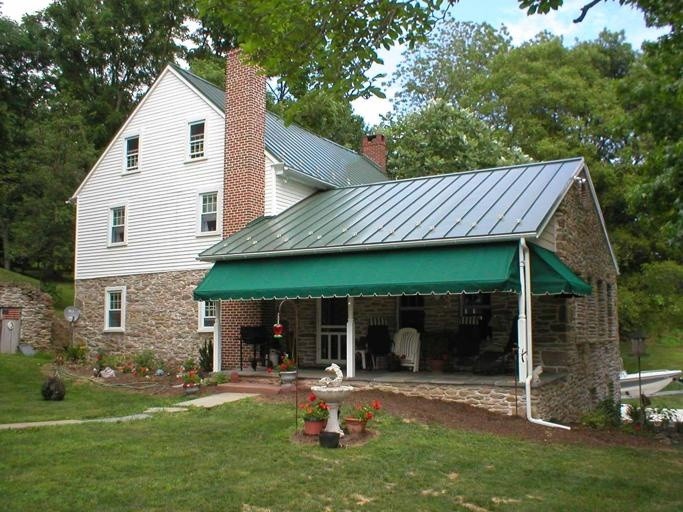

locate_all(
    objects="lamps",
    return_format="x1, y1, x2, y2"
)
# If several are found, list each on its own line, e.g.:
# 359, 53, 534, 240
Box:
273, 298, 300, 431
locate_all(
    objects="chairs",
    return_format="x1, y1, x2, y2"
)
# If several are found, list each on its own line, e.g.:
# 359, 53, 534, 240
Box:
346, 317, 422, 374
474, 314, 517, 377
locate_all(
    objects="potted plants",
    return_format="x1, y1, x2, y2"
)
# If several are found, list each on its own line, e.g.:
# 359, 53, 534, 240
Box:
425, 334, 449, 373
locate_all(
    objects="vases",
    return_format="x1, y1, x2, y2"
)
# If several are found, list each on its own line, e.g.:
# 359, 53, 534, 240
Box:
279, 371, 296, 380
319, 430, 340, 448
303, 421, 326, 437
343, 416, 367, 432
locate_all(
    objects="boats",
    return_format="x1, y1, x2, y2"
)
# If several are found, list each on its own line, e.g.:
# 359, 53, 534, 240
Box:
618, 358, 682, 400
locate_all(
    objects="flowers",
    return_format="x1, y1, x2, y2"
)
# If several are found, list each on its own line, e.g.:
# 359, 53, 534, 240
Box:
299, 396, 331, 423
346, 399, 381, 422
66, 340, 238, 394
278, 351, 296, 373
